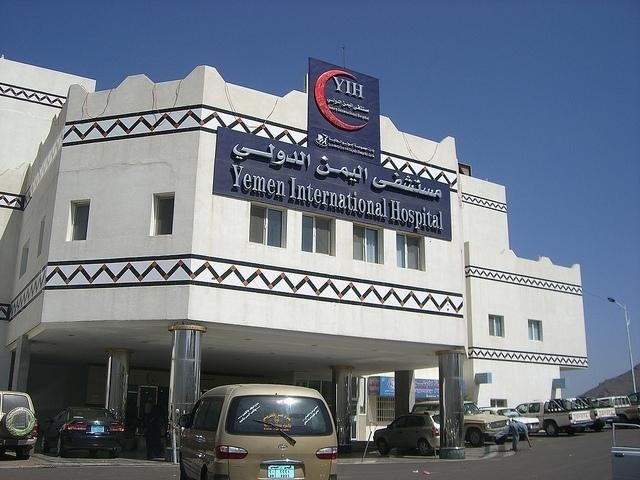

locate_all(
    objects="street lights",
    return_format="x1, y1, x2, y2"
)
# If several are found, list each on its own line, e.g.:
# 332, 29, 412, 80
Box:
607, 297, 638, 394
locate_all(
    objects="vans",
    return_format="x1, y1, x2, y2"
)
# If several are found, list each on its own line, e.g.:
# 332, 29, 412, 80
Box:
178, 384, 338, 480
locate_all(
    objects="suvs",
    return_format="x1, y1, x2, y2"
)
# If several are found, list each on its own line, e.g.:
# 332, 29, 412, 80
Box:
0, 391, 38, 459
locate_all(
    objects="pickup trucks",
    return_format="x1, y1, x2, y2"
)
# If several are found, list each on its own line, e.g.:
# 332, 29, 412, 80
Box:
513, 392, 640, 437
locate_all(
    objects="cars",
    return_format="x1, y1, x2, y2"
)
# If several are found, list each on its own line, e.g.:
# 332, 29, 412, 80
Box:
40, 406, 125, 459
611, 423, 640, 480
374, 400, 540, 455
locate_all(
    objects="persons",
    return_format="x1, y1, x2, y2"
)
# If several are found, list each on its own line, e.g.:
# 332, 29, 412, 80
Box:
496, 419, 532, 451
